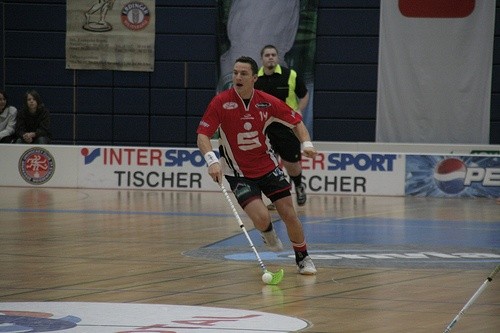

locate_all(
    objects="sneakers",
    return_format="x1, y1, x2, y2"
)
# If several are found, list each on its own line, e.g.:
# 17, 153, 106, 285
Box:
295, 189, 307, 206
259, 227, 284, 254
296, 256, 318, 274
267, 203, 276, 210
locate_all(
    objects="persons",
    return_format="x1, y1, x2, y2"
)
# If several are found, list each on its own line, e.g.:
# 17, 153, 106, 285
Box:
15, 91, 52, 144
253, 45, 309, 209
196, 56, 316, 275
0, 92, 18, 144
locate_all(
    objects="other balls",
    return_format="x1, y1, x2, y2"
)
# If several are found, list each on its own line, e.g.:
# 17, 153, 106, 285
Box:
261, 273, 272, 283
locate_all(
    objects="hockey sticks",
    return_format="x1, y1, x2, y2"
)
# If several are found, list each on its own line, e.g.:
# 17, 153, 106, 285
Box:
443, 264, 500, 332
216, 176, 284, 284
